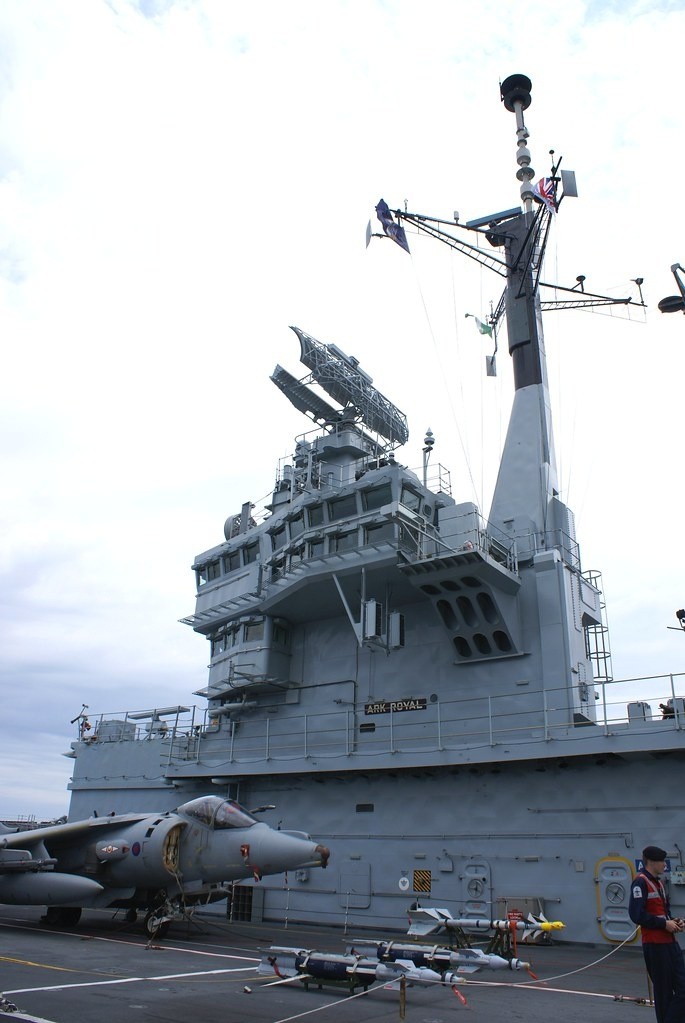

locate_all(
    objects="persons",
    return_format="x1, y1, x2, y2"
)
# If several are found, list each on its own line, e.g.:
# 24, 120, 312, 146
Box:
628, 846, 685, 1023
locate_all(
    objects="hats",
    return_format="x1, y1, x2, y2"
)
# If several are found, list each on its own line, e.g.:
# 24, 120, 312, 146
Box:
643, 846, 666, 861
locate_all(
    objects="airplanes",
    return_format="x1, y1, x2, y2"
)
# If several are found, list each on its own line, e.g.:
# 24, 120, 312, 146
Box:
0, 793, 329, 933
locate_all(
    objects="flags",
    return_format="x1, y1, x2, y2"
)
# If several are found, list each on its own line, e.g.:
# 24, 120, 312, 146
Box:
374, 197, 410, 254
532, 176, 556, 215
465, 313, 492, 338
